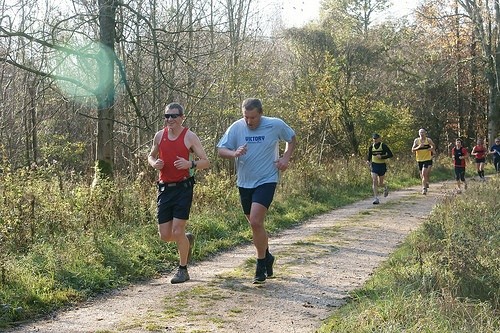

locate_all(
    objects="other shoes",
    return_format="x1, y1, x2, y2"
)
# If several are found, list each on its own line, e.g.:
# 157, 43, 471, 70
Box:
421, 187, 429, 195
372, 198, 379, 204
383, 187, 388, 197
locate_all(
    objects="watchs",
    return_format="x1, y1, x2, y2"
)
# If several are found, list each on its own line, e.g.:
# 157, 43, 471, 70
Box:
190, 161, 196, 170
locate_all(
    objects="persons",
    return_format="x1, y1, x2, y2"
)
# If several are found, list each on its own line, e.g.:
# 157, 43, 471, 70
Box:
147, 102, 209, 283
367, 133, 393, 205
452, 139, 469, 193
217, 98, 296, 283
490, 137, 500, 174
472, 139, 488, 179
412, 129, 437, 195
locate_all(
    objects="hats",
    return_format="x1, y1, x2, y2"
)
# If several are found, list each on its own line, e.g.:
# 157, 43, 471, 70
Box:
373, 134, 380, 139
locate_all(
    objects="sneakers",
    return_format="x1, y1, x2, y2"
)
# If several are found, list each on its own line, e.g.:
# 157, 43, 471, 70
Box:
251, 259, 267, 284
265, 253, 275, 278
185, 233, 195, 259
171, 267, 190, 284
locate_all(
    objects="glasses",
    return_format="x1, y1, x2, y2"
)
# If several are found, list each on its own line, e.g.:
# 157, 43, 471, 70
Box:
495, 141, 500, 143
164, 114, 182, 118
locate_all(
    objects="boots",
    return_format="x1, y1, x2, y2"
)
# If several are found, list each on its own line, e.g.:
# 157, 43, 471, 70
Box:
478, 170, 484, 179
